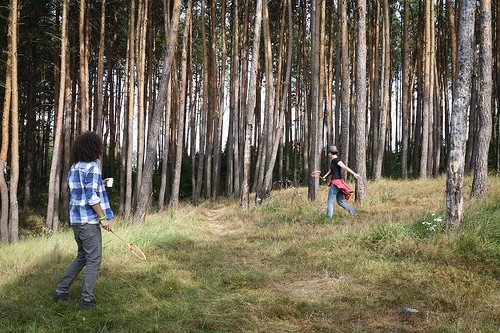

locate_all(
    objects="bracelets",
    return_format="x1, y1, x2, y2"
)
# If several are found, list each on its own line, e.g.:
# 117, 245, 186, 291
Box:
353, 172, 356, 175
99, 216, 106, 220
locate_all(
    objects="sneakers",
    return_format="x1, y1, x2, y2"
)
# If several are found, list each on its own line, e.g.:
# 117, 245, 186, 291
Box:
53, 292, 69, 301
79, 299, 96, 309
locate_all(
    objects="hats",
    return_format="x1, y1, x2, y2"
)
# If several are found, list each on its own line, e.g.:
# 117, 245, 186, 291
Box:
328, 146, 339, 152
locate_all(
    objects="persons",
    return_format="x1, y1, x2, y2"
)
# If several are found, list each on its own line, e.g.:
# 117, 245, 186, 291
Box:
322, 146, 361, 218
54, 131, 114, 307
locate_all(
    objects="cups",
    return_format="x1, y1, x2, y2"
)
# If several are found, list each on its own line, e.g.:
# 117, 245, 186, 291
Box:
106, 178, 113, 187
